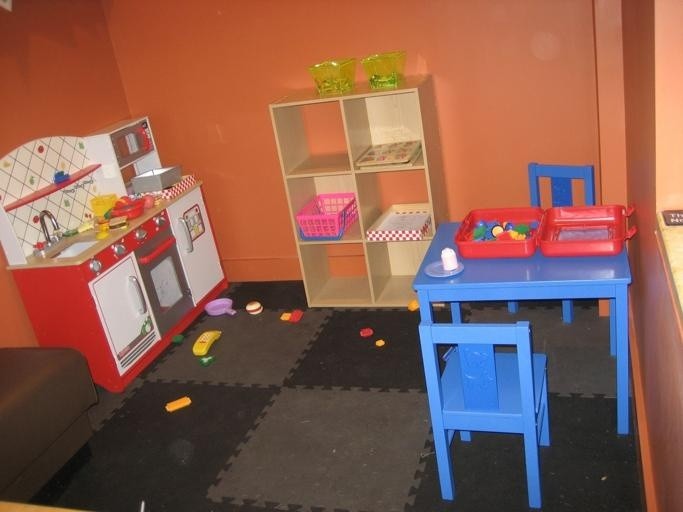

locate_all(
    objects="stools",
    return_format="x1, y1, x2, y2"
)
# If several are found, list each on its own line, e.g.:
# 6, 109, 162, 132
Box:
0, 345, 98, 503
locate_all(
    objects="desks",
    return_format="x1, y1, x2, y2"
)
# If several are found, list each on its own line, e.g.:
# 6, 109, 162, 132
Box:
410, 221, 633, 436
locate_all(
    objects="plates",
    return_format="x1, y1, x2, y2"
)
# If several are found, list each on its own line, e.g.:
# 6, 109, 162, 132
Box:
424, 261, 465, 279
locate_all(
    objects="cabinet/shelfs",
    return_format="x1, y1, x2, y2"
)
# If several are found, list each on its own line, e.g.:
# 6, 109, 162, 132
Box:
0, 115, 229, 394
267, 73, 437, 309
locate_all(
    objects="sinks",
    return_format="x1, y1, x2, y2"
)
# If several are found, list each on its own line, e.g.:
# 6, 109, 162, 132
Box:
48, 239, 100, 259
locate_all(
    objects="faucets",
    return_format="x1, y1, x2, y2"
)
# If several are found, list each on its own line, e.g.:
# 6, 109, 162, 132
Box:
38, 210, 60, 244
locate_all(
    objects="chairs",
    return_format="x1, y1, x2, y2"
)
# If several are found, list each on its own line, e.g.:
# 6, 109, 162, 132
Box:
505, 162, 596, 325
417, 320, 552, 509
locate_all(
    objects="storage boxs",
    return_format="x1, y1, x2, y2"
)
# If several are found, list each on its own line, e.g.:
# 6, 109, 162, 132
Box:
308, 50, 408, 97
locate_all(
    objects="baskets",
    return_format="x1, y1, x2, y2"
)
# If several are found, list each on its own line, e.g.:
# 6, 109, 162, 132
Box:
295, 192, 358, 241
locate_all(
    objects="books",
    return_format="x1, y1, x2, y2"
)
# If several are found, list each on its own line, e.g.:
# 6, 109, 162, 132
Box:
356, 141, 421, 166
359, 147, 422, 169
